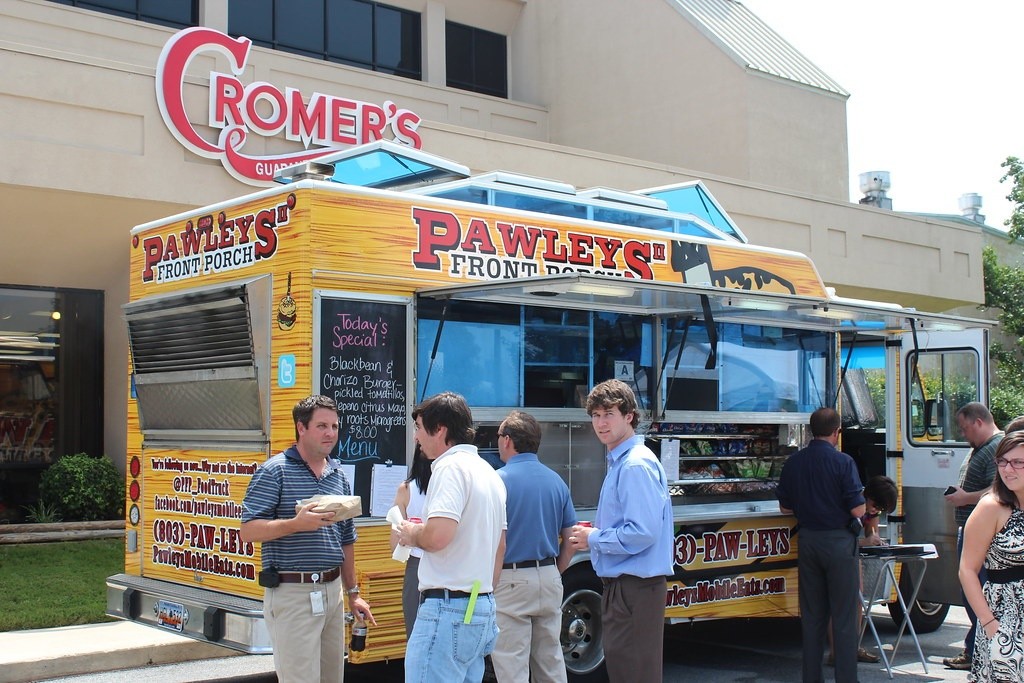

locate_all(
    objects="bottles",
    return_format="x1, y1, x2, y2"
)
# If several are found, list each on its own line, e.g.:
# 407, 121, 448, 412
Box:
348, 613, 367, 652
392, 518, 423, 563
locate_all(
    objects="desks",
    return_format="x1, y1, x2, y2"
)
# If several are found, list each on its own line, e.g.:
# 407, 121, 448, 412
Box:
857, 555, 935, 680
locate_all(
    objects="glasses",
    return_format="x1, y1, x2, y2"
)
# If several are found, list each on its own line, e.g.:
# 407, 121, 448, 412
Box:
992, 456, 1024, 473
834, 427, 843, 434
871, 504, 888, 512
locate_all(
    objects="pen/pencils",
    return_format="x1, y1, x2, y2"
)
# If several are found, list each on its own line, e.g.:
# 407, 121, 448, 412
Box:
872, 527, 875, 534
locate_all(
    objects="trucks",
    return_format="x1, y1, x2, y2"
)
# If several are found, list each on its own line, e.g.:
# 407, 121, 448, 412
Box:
98, 142, 991, 683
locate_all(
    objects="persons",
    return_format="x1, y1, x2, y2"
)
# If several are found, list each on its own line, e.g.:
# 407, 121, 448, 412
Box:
568, 380, 673, 683
390, 441, 433, 638
395, 392, 508, 683
957, 429, 1024, 683
241, 394, 377, 683
489, 411, 579, 683
828, 476, 898, 665
778, 408, 867, 683
1005, 416, 1024, 435
942, 402, 1005, 670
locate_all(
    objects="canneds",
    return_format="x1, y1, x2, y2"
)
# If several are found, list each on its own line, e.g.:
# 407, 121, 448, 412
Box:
576, 521, 593, 551
407, 517, 422, 524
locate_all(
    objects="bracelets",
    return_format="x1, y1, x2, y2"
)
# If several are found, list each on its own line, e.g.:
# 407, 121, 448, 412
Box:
984, 618, 995, 627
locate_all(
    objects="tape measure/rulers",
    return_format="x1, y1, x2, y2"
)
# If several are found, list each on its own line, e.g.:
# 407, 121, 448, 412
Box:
464, 580, 481, 624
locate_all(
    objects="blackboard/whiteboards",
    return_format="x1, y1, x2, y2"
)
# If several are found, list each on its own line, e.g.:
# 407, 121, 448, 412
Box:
311, 288, 416, 526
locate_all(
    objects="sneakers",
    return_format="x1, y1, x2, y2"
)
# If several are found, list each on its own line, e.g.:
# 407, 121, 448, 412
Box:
943, 648, 972, 670
827, 654, 835, 667
856, 646, 881, 663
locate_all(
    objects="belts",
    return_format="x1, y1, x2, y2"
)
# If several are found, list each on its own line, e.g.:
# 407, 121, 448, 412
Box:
422, 588, 492, 599
279, 567, 340, 584
600, 573, 629, 587
502, 558, 555, 569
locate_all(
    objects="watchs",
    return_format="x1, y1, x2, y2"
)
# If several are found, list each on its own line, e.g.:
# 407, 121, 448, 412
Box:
348, 586, 359, 594
